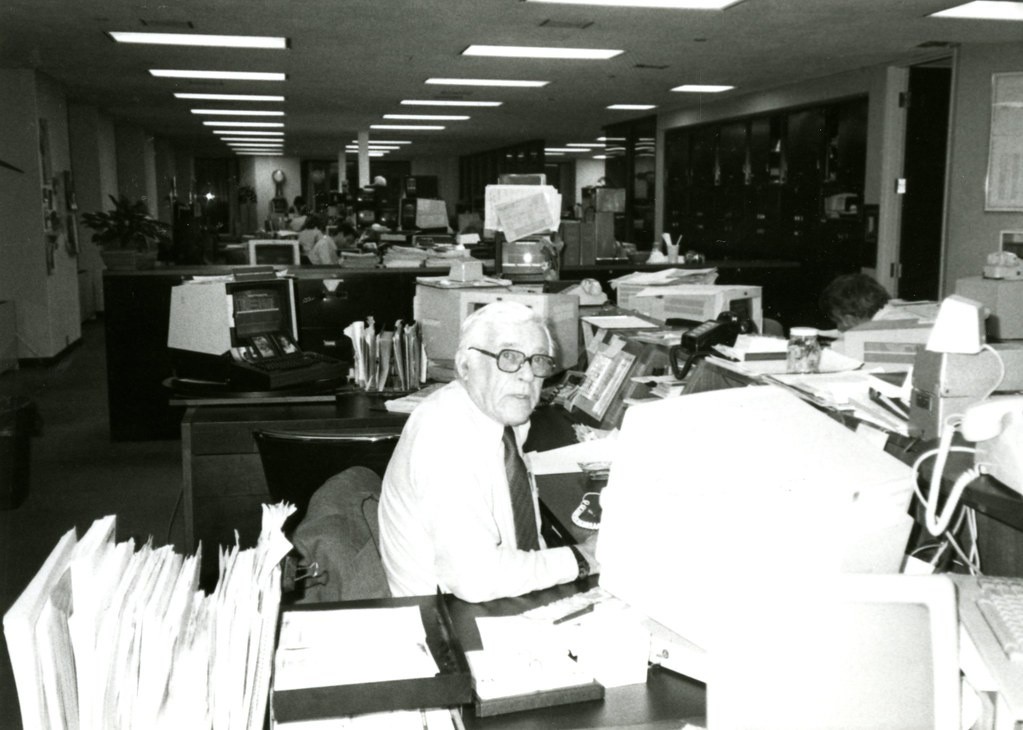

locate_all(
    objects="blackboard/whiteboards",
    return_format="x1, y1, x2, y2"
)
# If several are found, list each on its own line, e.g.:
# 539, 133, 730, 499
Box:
983, 70, 1023, 212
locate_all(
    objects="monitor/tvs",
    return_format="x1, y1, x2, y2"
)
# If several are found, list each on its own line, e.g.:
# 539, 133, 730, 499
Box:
593, 385, 957, 730
413, 231, 581, 385
175, 240, 301, 382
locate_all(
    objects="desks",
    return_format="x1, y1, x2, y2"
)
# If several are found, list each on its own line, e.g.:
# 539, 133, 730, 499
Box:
102, 225, 707, 730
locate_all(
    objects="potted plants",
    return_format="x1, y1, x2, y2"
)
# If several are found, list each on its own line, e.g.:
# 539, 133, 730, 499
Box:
79, 193, 178, 271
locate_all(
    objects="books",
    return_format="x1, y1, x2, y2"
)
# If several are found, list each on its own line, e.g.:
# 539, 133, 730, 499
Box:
2, 498, 294, 730
705, 334, 917, 438
342, 317, 430, 392
340, 243, 471, 268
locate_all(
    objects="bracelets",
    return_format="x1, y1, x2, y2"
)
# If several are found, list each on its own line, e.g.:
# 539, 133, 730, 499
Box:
570, 546, 590, 581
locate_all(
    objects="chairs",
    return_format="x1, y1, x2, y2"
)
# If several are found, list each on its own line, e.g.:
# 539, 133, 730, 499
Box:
250, 426, 400, 536
291, 464, 391, 604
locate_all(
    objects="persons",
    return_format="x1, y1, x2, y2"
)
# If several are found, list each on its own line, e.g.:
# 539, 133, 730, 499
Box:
299, 214, 320, 251
190, 203, 221, 264
302, 216, 338, 265
379, 301, 599, 606
822, 274, 891, 333
310, 218, 350, 266
287, 195, 314, 230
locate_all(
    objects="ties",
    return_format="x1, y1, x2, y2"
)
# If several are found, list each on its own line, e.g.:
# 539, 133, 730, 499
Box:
502, 425, 541, 552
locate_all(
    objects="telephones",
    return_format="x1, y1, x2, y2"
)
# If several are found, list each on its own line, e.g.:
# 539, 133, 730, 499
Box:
680, 308, 756, 359
925, 396, 1023, 538
982, 251, 1023, 281
558, 278, 609, 305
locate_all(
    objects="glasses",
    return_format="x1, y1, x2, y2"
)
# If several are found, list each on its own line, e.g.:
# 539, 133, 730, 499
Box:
467, 347, 557, 378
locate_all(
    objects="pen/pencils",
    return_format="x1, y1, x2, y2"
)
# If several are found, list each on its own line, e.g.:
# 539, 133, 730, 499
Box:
674, 233, 685, 250
549, 597, 602, 625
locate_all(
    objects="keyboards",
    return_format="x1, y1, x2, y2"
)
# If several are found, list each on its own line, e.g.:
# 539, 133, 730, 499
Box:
520, 586, 620, 630
950, 570, 1023, 721
